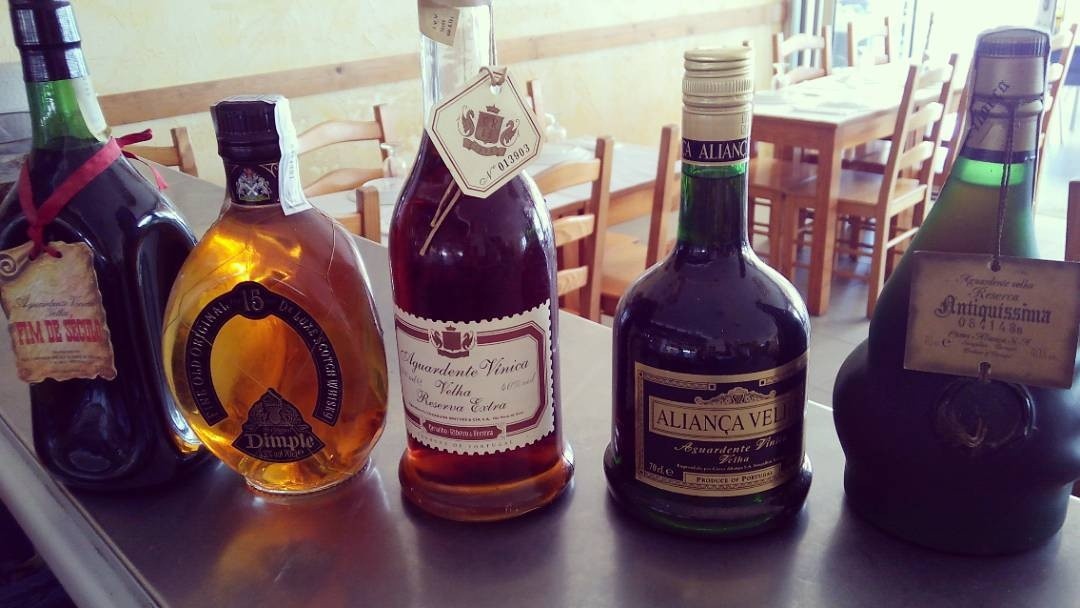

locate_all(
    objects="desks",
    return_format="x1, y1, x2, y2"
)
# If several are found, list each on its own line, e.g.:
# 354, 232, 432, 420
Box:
675, 66, 976, 316
305, 135, 681, 327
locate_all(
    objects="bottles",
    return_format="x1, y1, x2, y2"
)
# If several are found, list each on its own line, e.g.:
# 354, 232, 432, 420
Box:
831, 23, 1079, 554
0, 2, 227, 494
602, 39, 814, 540
387, 0, 580, 523
161, 93, 387, 496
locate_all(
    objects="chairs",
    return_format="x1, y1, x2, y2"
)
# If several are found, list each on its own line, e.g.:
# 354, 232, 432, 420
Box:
124, 17, 1080, 327
